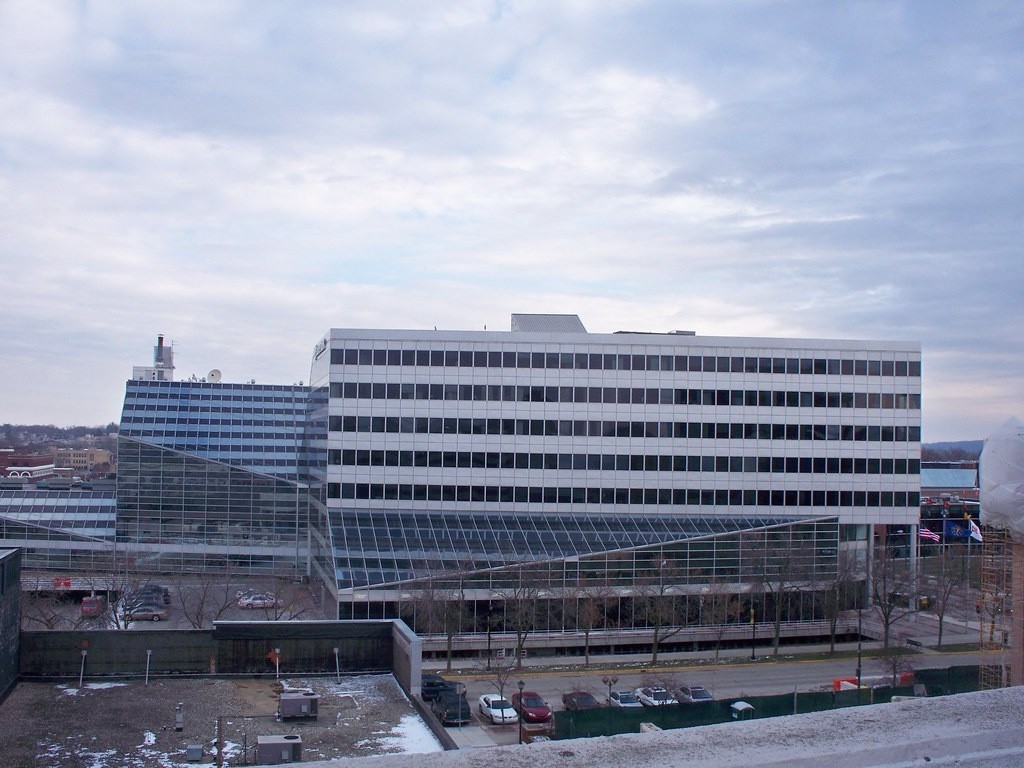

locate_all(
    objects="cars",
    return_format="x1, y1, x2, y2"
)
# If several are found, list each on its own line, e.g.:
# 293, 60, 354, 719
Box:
512, 692, 552, 723
634, 687, 679, 705
604, 690, 643, 708
119, 606, 168, 622
675, 687, 714, 702
237, 589, 280, 600
561, 692, 602, 710
238, 595, 284, 609
122, 585, 170, 610
479, 694, 518, 724
977, 593, 1013, 614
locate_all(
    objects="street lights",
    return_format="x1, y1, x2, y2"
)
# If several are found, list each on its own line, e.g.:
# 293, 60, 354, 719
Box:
517, 680, 526, 744
602, 675, 618, 735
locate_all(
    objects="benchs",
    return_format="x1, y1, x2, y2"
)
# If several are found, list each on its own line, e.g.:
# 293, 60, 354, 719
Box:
906, 639, 923, 650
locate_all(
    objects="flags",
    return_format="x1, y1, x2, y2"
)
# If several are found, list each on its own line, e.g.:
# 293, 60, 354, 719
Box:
944, 521, 972, 544
969, 519, 982, 542
919, 524, 940, 542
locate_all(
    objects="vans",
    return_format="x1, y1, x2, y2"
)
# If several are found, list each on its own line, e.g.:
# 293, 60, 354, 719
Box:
888, 586, 937, 610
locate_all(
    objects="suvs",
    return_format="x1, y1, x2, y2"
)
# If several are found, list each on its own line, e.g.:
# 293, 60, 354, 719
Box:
81, 599, 104, 618
421, 675, 466, 700
432, 691, 471, 726
